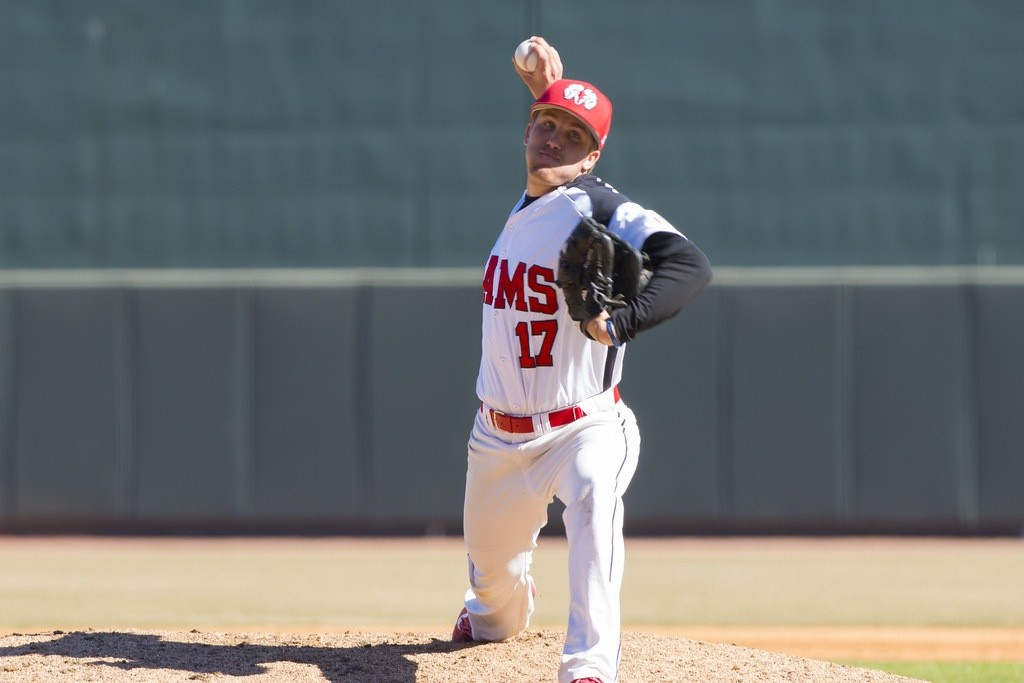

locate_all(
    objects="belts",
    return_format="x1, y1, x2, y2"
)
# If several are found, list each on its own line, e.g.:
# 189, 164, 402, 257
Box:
481, 386, 620, 433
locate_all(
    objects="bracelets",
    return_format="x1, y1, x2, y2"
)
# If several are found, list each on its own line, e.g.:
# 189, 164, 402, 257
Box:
606, 317, 622, 348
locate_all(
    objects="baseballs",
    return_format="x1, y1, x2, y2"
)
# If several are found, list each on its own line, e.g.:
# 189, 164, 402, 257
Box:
516, 39, 539, 74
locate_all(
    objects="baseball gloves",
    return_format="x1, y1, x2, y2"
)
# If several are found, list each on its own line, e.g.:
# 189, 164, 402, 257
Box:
557, 217, 651, 341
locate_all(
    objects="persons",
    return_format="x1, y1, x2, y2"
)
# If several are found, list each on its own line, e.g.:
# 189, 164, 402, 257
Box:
446, 37, 714, 683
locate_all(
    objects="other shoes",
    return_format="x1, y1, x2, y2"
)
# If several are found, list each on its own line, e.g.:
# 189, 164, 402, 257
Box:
452, 607, 473, 644
570, 677, 604, 683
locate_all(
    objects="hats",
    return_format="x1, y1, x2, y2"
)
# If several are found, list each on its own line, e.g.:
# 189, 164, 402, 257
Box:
530, 79, 612, 151
